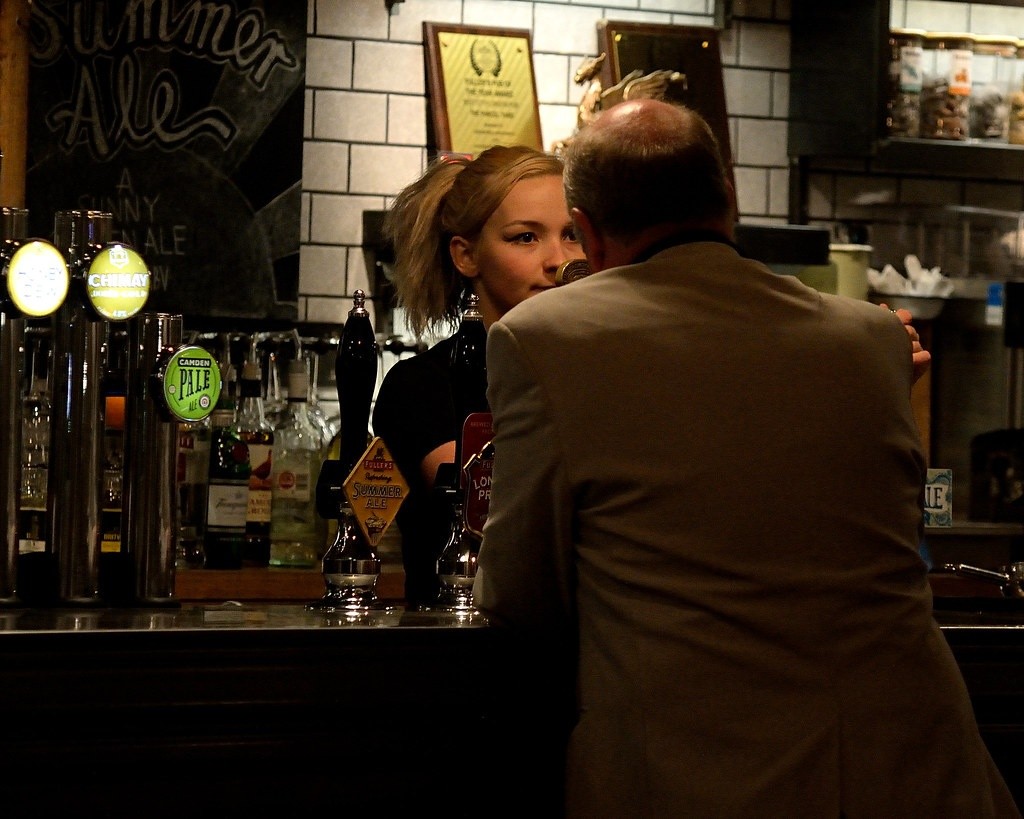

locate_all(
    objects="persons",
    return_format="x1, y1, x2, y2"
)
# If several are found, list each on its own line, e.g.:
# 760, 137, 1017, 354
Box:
470, 98, 1023, 819
372, 146, 931, 609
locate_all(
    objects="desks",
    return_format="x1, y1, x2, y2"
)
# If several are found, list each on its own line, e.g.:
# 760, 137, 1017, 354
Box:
0, 567, 1024, 819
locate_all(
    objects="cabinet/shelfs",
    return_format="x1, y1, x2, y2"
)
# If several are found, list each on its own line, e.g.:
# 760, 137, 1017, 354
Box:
778, 138, 1022, 562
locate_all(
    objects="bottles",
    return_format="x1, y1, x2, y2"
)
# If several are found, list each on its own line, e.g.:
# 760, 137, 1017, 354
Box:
890, 27, 1023, 145
2, 208, 483, 617
